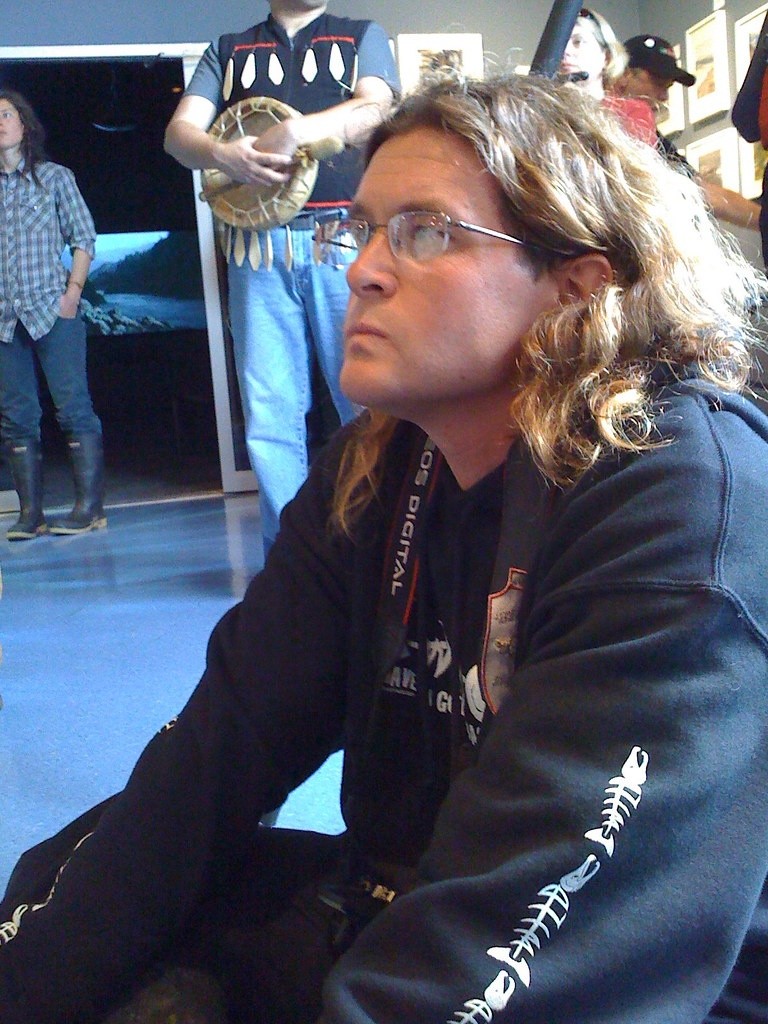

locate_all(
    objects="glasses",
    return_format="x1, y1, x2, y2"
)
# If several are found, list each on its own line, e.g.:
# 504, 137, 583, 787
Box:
311, 206, 580, 268
577, 6, 601, 24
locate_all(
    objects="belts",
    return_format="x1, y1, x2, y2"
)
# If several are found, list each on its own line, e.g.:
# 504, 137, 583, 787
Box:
286, 208, 347, 232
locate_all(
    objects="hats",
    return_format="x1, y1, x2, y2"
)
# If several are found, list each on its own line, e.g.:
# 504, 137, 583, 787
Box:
622, 35, 697, 86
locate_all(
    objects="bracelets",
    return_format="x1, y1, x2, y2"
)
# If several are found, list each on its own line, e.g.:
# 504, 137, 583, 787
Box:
67, 279, 84, 289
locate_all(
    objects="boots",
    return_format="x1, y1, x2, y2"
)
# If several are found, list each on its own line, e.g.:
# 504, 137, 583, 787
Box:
3, 435, 48, 540
49, 433, 107, 534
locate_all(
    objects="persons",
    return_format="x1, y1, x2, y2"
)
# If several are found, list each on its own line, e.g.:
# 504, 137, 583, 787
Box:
553, 10, 657, 147
605, 36, 761, 232
1, 72, 768, 1024
0, 90, 112, 535
159, 0, 404, 552
732, 13, 768, 269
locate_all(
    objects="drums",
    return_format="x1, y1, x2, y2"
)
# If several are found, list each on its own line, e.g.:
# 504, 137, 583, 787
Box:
199, 95, 320, 231
